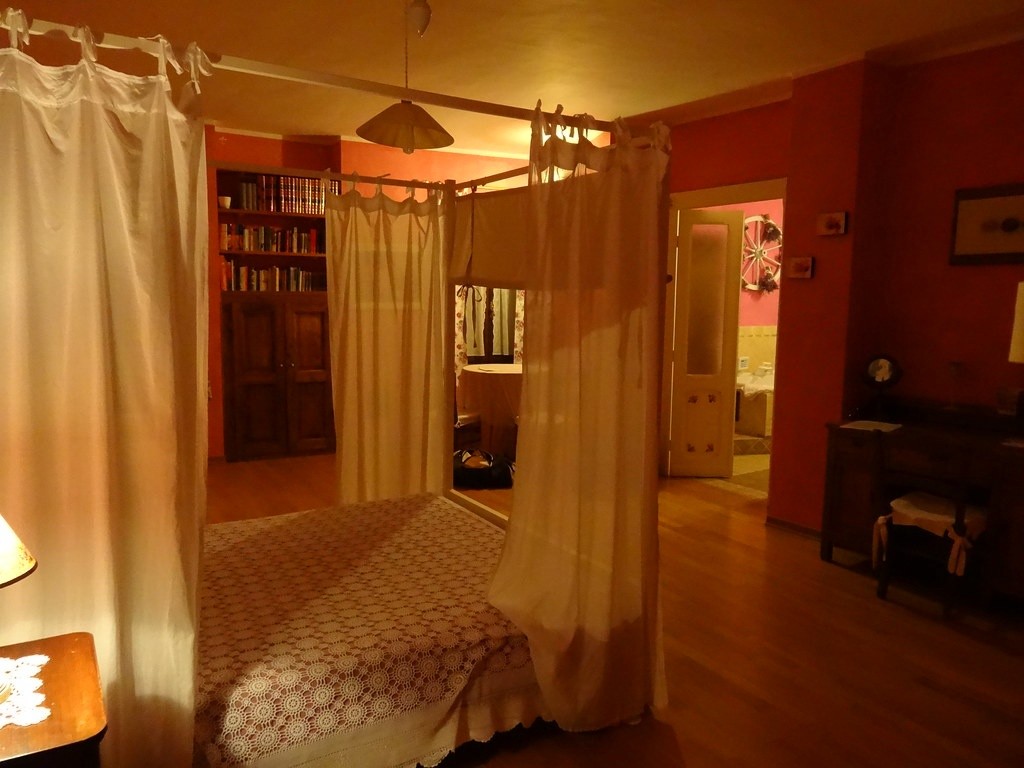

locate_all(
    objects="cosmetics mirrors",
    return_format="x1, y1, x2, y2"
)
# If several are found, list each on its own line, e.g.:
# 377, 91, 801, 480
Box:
863, 354, 903, 386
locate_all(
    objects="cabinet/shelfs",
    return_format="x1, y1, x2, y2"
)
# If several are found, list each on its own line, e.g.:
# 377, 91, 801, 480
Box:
206, 160, 342, 464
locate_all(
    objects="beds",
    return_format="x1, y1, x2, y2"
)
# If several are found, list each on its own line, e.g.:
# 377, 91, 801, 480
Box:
0, 7, 674, 768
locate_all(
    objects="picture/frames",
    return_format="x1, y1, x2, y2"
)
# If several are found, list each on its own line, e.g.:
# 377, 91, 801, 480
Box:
947, 181, 1024, 266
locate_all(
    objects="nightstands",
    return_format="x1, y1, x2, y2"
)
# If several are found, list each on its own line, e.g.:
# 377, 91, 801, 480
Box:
0, 632, 108, 768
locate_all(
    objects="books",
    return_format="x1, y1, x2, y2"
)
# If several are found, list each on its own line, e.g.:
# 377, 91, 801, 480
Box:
232, 176, 339, 215
219, 223, 317, 254
218, 254, 312, 292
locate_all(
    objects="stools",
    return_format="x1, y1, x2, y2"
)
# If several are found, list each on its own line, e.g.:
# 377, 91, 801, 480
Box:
872, 490, 989, 621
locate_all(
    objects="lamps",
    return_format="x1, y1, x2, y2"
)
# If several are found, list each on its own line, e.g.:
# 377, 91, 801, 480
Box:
356, 0, 454, 154
0, 513, 38, 587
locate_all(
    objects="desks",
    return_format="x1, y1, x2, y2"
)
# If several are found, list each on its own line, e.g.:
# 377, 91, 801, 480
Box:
819, 400, 1024, 599
456, 364, 522, 459
